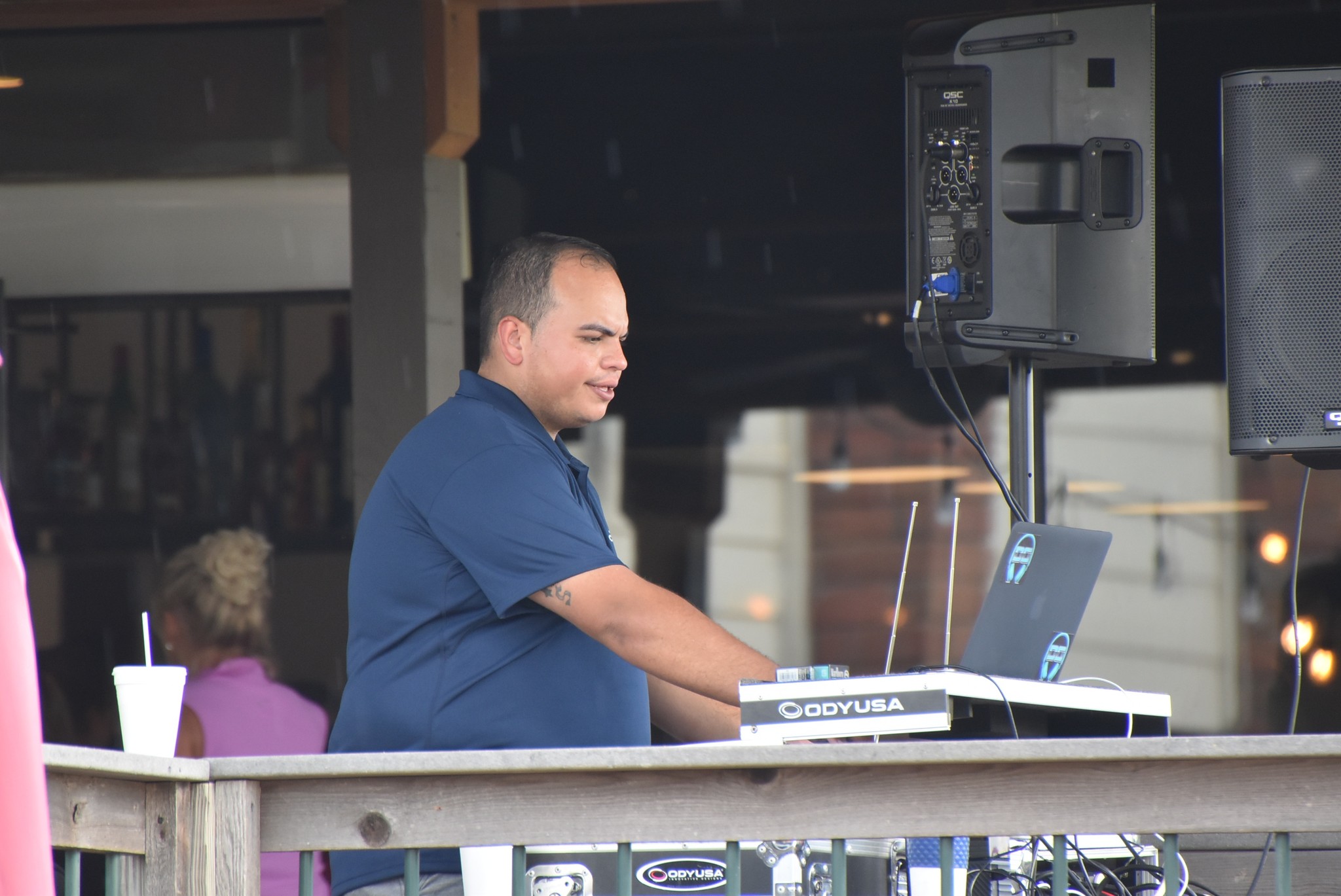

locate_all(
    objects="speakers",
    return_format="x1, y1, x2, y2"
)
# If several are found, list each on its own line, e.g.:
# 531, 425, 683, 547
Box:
1216, 66, 1341, 460
905, 1, 1157, 371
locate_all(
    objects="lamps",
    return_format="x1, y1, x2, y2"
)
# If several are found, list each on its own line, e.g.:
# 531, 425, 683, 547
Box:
1279, 614, 1333, 683
1250, 527, 1288, 564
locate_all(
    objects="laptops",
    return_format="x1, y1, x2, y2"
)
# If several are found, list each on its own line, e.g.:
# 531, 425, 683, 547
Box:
959, 522, 1111, 684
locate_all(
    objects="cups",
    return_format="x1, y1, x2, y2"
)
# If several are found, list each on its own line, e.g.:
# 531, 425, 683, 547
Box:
111, 666, 188, 758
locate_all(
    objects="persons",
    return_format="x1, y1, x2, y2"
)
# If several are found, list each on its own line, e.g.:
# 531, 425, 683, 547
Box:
328, 233, 781, 896
157, 529, 334, 896
